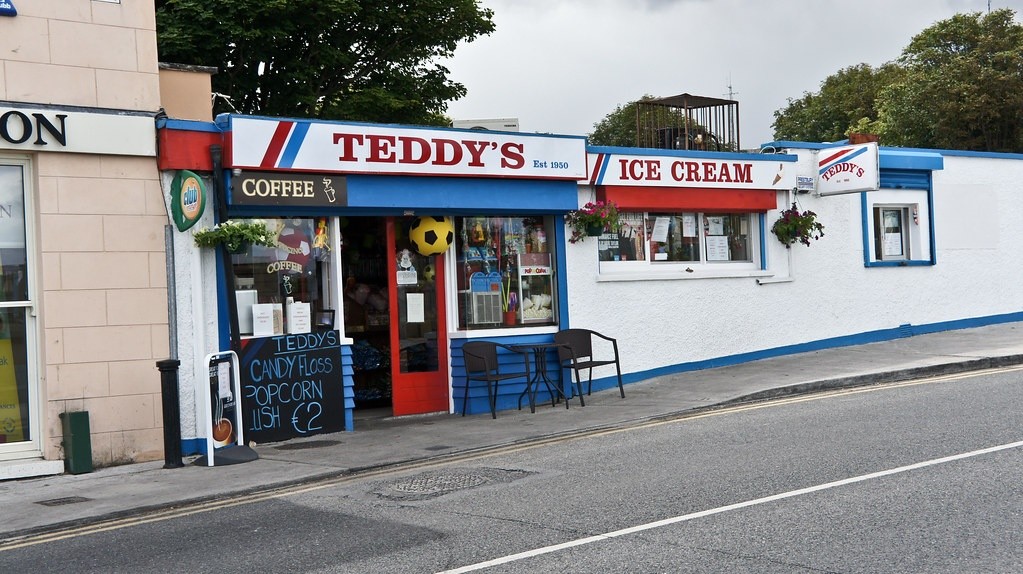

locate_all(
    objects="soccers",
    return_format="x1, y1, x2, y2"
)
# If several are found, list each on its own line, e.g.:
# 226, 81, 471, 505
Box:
422, 263, 435, 287
408, 215, 454, 258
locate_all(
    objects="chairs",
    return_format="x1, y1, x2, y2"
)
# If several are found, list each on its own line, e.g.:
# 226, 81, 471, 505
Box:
461, 341, 534, 419
554, 329, 625, 408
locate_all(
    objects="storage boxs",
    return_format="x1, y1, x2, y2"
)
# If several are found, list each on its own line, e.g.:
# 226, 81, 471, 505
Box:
252, 303, 283, 336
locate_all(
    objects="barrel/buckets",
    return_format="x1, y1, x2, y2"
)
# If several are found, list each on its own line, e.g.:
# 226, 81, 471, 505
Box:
504, 312, 516, 326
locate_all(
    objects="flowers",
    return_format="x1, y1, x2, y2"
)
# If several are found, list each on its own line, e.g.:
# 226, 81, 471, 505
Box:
770, 202, 825, 249
567, 199, 629, 244
191, 219, 280, 251
522, 217, 543, 234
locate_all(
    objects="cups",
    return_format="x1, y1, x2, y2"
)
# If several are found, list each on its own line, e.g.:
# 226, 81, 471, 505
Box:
212, 418, 232, 449
523, 294, 551, 310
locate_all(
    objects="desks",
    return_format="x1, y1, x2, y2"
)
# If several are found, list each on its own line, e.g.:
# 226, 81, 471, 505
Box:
511, 343, 571, 413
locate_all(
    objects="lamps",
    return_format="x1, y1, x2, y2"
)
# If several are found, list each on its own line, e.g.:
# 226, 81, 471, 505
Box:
313, 309, 335, 331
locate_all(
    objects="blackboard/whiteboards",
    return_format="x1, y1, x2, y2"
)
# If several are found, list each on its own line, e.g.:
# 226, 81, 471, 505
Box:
238, 329, 346, 446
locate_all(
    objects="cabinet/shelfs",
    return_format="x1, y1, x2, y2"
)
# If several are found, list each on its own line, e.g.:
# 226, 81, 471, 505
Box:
516, 251, 556, 325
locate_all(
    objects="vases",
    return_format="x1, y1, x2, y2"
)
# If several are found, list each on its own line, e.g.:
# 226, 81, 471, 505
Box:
229, 240, 253, 255
585, 222, 604, 236
532, 225, 542, 231
791, 230, 801, 239
